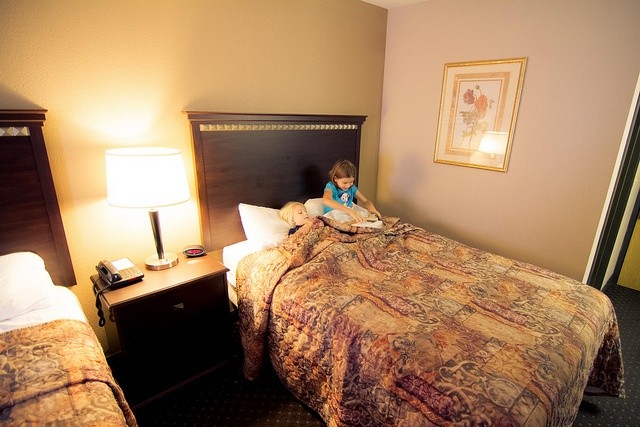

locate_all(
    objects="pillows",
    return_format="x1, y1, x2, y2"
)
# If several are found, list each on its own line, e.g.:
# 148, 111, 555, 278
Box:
0, 250, 61, 322
239, 202, 296, 245
304, 198, 325, 218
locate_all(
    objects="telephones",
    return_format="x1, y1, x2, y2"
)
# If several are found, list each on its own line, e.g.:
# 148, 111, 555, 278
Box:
93, 256, 144, 286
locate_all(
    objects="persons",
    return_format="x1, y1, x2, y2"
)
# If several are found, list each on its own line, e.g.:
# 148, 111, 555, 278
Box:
280, 202, 310, 239
322, 160, 382, 223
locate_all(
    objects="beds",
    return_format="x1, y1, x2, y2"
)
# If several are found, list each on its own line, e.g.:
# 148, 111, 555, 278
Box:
0, 109, 141, 427
184, 113, 625, 427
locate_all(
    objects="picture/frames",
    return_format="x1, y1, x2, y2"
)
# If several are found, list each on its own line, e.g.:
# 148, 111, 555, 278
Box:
433, 57, 531, 173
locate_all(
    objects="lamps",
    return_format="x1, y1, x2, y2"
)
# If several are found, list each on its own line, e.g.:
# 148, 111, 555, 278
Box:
104, 146, 191, 270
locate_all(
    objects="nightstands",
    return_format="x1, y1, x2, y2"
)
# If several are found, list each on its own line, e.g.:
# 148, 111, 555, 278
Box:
90, 253, 232, 407
